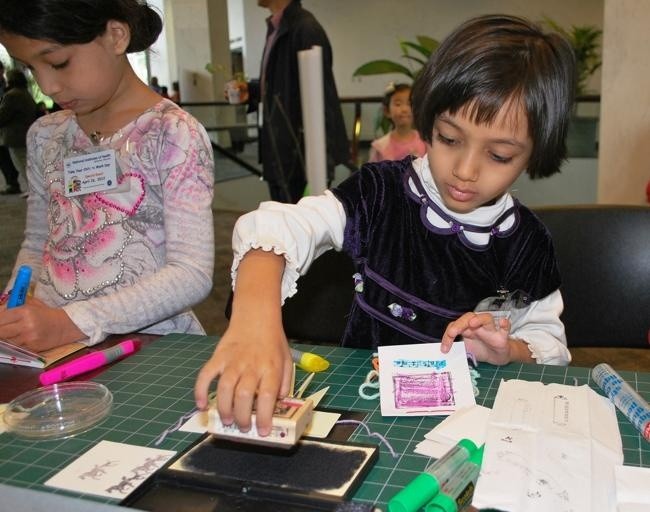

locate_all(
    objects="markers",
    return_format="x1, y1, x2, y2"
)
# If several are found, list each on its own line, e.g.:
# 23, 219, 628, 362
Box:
424, 441, 486, 512
388, 438, 478, 512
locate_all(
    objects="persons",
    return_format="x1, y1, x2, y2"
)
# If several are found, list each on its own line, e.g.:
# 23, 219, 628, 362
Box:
0, 0, 217, 353
0, 61, 21, 196
194, 16, 580, 436
0, 69, 44, 199
222, 0, 351, 205
369, 83, 426, 162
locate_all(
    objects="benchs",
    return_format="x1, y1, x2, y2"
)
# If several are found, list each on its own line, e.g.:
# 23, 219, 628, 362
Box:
225, 205, 650, 346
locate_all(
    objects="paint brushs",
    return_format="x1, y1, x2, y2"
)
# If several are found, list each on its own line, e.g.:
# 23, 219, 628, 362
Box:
288, 344, 331, 373
39, 338, 144, 388
6, 265, 33, 311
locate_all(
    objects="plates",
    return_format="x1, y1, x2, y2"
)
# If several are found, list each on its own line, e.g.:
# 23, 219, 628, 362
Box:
3, 381, 113, 442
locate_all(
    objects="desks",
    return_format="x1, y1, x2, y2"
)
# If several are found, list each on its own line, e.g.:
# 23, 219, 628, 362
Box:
1, 332, 650, 512
